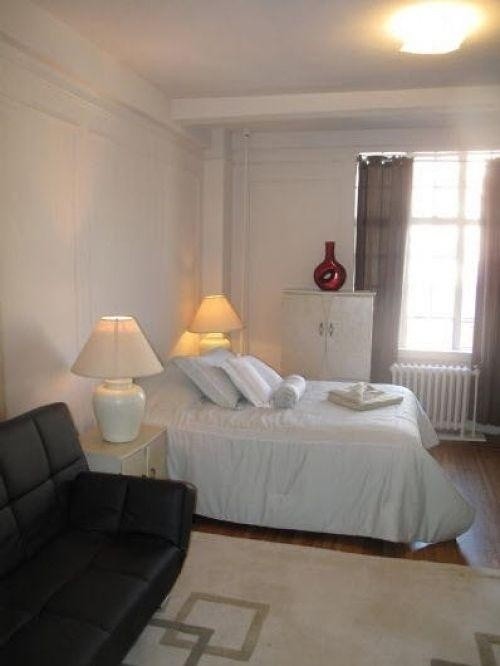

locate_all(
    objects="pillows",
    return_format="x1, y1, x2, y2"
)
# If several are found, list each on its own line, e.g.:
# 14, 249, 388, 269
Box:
135, 360, 205, 406
219, 354, 285, 408
170, 345, 243, 410
273, 374, 307, 409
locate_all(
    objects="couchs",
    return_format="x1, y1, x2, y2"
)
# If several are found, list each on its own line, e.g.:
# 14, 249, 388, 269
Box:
1, 401, 198, 665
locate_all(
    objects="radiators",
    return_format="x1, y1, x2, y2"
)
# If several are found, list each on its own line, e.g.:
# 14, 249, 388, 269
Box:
389, 363, 489, 442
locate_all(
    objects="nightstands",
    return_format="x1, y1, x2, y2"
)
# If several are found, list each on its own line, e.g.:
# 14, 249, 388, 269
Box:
78, 422, 169, 480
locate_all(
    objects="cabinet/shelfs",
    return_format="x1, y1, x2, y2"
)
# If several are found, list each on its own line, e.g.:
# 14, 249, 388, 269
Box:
279, 289, 377, 383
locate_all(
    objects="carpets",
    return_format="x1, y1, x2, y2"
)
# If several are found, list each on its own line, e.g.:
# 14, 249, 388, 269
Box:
118, 531, 499, 664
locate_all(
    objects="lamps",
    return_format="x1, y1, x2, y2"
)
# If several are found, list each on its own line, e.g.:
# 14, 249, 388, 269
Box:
187, 295, 245, 353
70, 314, 165, 444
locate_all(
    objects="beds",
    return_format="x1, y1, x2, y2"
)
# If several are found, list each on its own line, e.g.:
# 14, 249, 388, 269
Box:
136, 377, 476, 547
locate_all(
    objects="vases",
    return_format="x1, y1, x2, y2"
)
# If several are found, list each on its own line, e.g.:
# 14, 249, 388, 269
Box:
314, 240, 347, 291
